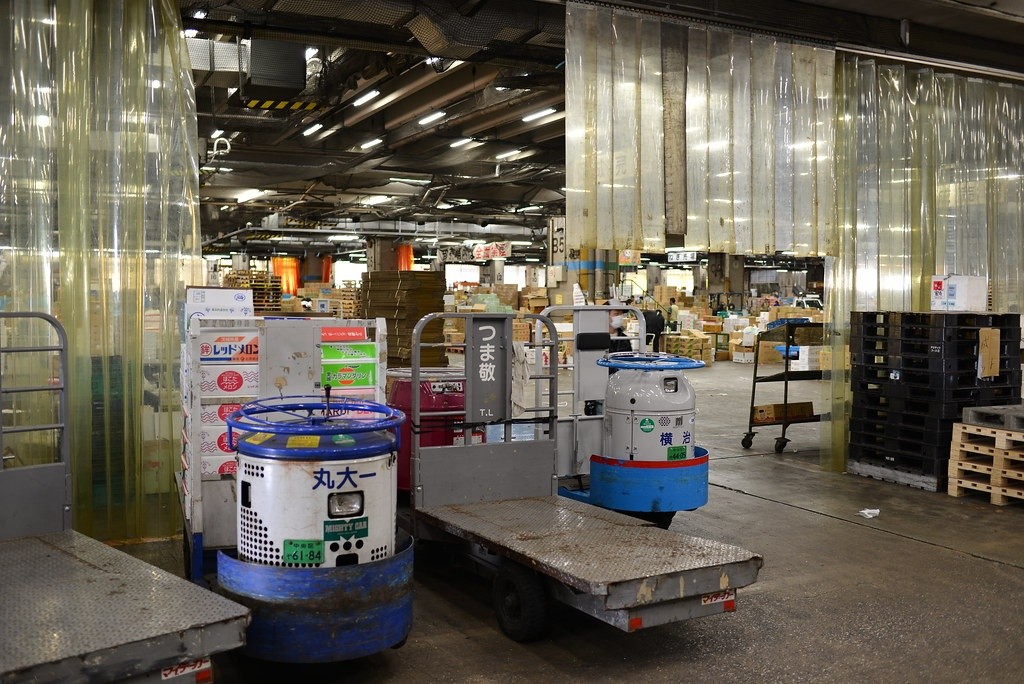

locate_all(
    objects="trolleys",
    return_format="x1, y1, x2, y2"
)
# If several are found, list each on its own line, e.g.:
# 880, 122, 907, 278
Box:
488, 303, 649, 480
0, 310, 253, 684
395, 311, 766, 642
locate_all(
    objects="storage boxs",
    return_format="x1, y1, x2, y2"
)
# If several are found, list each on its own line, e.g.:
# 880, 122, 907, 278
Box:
178, 283, 378, 518
751, 401, 813, 423
620, 281, 852, 371
442, 283, 574, 371
930, 276, 988, 311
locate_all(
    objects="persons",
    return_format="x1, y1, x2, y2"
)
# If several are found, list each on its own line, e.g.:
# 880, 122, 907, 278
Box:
602, 299, 630, 375
301, 297, 314, 311
626, 299, 634, 306
631, 295, 643, 304
643, 309, 664, 353
667, 297, 679, 324
774, 301, 781, 306
761, 299, 770, 309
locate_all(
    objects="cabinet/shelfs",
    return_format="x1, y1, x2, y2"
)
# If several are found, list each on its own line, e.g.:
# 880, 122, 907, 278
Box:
741, 322, 852, 453
